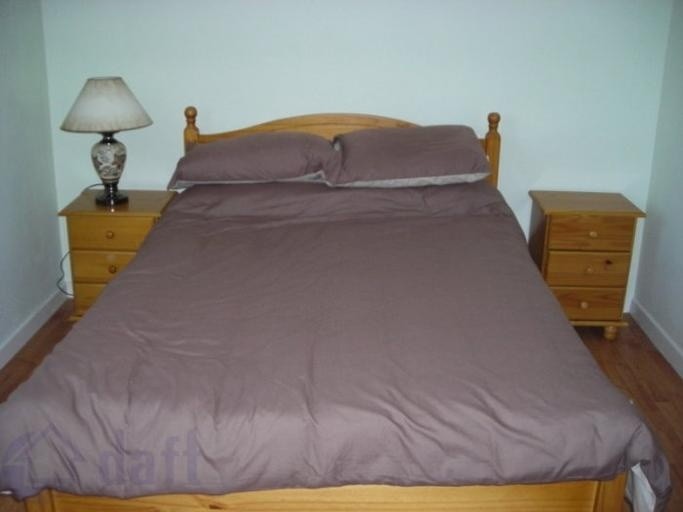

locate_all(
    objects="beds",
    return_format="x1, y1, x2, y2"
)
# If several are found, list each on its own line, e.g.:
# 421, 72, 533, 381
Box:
0, 108, 683, 512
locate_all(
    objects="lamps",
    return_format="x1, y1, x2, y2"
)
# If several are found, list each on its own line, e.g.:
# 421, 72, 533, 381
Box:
58, 72, 155, 207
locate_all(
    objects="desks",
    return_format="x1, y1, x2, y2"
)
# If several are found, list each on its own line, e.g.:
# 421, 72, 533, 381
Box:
527, 188, 646, 344
57, 187, 177, 324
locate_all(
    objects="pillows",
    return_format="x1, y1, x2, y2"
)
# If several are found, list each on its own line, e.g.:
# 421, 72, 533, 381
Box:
331, 120, 492, 193
164, 131, 331, 192
